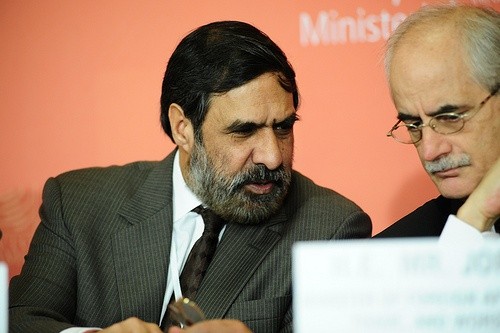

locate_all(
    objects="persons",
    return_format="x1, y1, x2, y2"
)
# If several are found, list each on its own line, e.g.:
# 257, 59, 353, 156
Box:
439, 157, 500, 237
369, 3, 500, 237
7, 21, 373, 333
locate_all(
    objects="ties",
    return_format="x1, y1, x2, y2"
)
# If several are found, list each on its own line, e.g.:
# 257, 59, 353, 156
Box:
158, 204, 226, 331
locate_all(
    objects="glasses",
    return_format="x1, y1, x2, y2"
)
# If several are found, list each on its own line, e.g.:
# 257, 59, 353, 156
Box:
386, 86, 499, 145
169, 298, 206, 325
